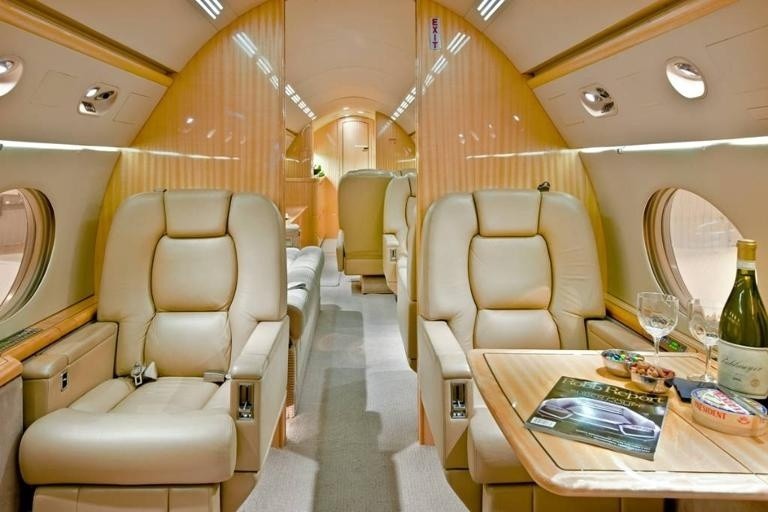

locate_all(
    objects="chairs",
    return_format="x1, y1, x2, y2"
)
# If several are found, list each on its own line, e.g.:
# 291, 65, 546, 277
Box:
17, 189, 290, 511
336, 170, 423, 371
416, 187, 661, 512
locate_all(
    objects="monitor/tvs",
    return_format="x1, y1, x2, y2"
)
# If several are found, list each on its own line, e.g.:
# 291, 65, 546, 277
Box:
522, 375, 669, 462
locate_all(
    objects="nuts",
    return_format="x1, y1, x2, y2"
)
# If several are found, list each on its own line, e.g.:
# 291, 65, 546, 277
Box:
630, 360, 676, 380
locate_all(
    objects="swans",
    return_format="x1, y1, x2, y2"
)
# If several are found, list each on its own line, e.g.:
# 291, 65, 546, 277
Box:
603, 349, 645, 363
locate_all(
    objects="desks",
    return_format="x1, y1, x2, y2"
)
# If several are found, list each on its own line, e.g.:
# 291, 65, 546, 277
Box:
465, 348, 767, 505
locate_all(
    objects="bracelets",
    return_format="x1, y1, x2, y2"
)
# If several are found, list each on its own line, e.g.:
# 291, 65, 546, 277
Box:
600, 349, 645, 378
630, 368, 676, 393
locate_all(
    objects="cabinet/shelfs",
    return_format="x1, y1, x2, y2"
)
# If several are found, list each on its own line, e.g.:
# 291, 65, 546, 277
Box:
719, 239, 768, 416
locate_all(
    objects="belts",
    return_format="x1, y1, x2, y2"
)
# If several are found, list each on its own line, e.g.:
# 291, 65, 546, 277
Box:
687, 297, 725, 389
636, 292, 680, 370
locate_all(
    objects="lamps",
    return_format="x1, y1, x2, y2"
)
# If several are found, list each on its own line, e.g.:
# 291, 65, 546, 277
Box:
17, 189, 290, 511
